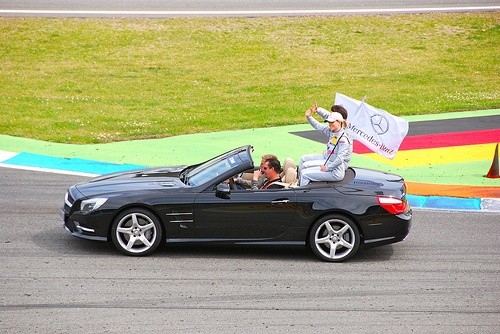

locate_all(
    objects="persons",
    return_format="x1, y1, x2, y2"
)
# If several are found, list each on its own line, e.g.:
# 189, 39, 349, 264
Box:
258, 154, 277, 178
296, 107, 350, 187
227, 158, 284, 190
297, 99, 352, 169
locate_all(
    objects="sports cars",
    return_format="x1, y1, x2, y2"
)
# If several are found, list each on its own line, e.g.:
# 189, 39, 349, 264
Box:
57, 144, 413, 263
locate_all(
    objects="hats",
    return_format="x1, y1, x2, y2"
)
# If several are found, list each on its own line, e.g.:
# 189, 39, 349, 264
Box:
324, 112, 344, 123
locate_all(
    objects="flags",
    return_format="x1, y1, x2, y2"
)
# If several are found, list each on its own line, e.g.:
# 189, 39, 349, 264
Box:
344, 99, 409, 160
334, 92, 360, 122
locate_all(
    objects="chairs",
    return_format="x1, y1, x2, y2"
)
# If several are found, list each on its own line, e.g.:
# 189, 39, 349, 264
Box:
281, 167, 297, 183
279, 157, 295, 177
